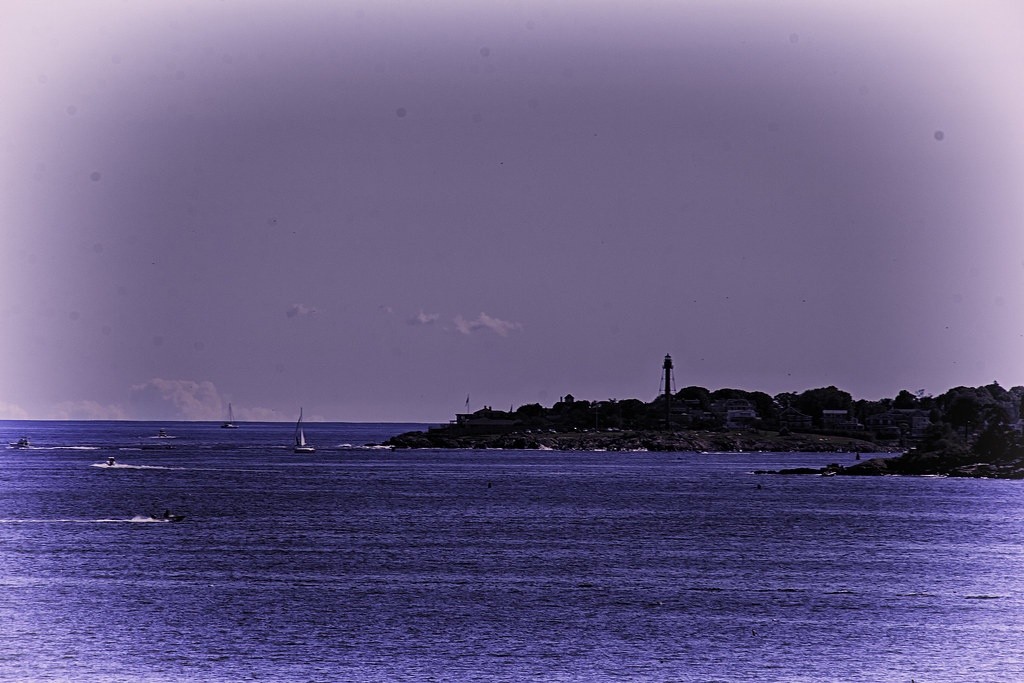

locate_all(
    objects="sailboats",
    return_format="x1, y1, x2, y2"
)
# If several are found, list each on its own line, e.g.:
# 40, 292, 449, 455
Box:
219, 402, 240, 429
292, 407, 318, 453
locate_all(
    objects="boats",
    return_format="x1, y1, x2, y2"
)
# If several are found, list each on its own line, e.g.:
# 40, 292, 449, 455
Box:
151, 510, 186, 523
106, 456, 116, 465
158, 428, 168, 439
10, 436, 31, 448
